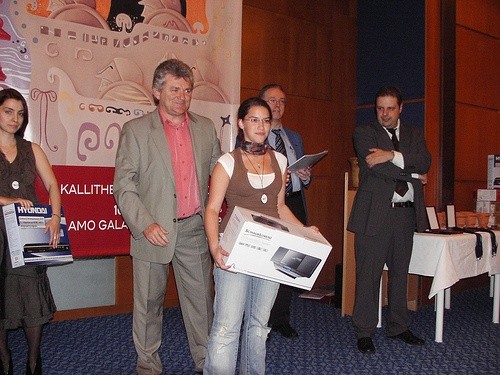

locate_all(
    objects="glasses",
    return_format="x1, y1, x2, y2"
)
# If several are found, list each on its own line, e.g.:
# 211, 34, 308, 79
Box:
243, 117, 272, 125
264, 98, 288, 106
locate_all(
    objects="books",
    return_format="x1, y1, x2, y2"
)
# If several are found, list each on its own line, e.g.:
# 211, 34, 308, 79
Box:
287, 151, 328, 174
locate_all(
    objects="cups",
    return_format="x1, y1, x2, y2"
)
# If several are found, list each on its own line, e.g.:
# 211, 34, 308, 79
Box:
466, 212, 478, 229
478, 213, 490, 229
454, 212, 466, 229
437, 212, 448, 230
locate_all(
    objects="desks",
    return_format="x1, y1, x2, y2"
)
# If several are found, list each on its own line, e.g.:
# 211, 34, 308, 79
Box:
375, 230, 500, 344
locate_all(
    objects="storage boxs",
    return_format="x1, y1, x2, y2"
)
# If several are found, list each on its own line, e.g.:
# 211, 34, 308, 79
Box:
219, 206, 332, 291
477, 154, 500, 230
2, 203, 73, 269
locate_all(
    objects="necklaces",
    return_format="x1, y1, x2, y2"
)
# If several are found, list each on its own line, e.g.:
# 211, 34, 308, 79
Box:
253, 158, 262, 168
243, 151, 268, 204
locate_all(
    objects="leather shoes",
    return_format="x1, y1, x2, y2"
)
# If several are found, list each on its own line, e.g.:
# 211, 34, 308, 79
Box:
357, 337, 375, 354
390, 329, 424, 345
271, 323, 299, 338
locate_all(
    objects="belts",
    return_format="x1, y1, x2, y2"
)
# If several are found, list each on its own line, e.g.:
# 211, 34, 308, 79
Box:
391, 202, 415, 207
293, 190, 302, 197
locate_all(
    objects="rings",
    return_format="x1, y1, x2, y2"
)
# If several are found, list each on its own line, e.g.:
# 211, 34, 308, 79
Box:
54, 237, 56, 239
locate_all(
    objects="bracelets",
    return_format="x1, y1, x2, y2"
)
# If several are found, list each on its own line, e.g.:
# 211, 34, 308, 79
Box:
51, 213, 61, 220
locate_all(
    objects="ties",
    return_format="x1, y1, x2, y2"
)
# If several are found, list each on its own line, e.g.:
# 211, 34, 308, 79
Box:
271, 128, 293, 199
387, 127, 409, 197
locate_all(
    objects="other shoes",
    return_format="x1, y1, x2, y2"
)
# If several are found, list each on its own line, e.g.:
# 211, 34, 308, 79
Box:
0, 357, 12, 375
26, 356, 39, 375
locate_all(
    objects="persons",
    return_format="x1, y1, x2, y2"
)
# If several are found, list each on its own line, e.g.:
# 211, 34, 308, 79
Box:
346, 86, 432, 353
115, 58, 222, 375
0, 88, 61, 375
203, 98, 319, 375
258, 83, 312, 342
